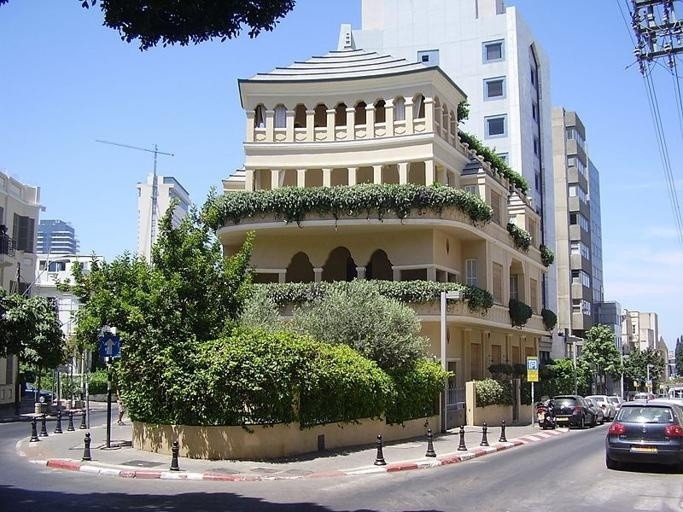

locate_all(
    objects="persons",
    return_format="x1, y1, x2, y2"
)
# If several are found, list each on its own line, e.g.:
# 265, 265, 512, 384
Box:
115, 390, 126, 423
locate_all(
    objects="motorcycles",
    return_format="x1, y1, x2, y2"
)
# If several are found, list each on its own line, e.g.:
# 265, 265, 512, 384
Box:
535, 398, 554, 429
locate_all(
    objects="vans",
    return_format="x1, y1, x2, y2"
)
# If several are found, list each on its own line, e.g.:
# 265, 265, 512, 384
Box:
553, 393, 615, 429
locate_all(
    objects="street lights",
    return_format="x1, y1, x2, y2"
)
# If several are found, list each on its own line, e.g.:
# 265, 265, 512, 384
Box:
11, 258, 75, 413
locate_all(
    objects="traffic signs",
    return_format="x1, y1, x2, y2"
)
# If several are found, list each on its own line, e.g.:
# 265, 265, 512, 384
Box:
525, 356, 538, 383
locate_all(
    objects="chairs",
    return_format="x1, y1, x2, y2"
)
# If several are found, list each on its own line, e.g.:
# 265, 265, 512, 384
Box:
625, 408, 671, 421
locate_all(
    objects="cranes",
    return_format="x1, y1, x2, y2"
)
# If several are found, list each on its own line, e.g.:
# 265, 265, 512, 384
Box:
98, 140, 174, 263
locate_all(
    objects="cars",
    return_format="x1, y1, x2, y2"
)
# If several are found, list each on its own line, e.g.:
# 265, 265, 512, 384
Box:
604, 385, 683, 470
